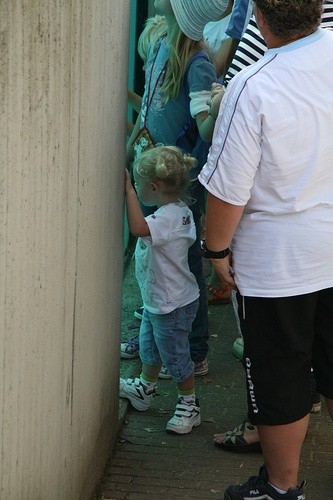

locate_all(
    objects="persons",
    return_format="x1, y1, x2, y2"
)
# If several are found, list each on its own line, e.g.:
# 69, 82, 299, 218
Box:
208, 0, 333, 454
196, 0, 332, 500
119, 0, 333, 412
119, 145, 206, 438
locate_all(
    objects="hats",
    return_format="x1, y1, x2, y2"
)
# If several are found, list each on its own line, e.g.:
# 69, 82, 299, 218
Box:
169, 0, 230, 40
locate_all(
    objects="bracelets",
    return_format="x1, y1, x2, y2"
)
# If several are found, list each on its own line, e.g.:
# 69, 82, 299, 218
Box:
207, 108, 218, 119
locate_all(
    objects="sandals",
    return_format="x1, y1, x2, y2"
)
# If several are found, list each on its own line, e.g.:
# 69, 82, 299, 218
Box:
207, 282, 231, 304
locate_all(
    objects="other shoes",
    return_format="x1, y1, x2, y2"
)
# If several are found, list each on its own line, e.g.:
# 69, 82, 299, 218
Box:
121, 335, 140, 359
158, 357, 209, 378
133, 307, 144, 319
224, 461, 306, 500
214, 419, 262, 453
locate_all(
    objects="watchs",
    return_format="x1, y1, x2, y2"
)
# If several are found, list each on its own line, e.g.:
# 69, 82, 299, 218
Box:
199, 238, 230, 258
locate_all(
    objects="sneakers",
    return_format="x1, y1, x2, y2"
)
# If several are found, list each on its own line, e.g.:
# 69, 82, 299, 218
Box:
120, 377, 156, 411
166, 397, 201, 435
